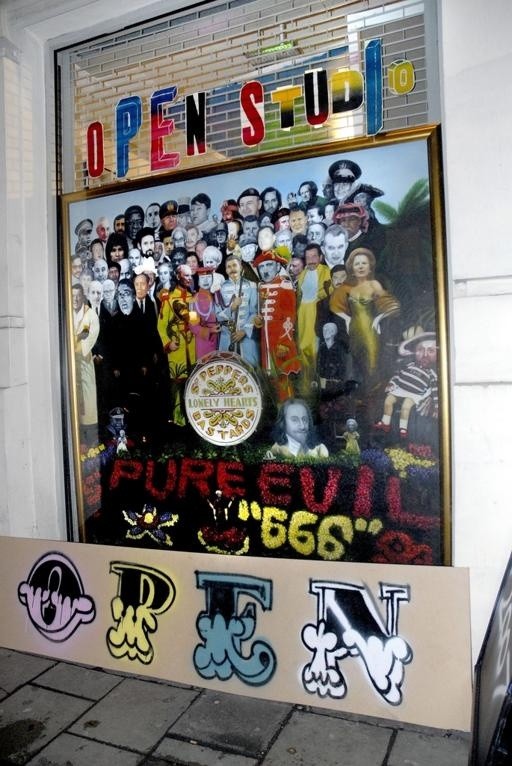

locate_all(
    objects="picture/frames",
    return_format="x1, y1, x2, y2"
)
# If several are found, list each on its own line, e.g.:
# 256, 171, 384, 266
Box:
55, 121, 454, 568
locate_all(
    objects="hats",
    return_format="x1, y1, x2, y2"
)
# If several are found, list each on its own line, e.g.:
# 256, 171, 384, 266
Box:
237, 187, 259, 203
403, 331, 437, 353
159, 201, 177, 218
340, 183, 385, 206
75, 219, 93, 236
271, 208, 290, 224
216, 222, 228, 234
252, 249, 288, 267
329, 160, 361, 182
196, 267, 214, 276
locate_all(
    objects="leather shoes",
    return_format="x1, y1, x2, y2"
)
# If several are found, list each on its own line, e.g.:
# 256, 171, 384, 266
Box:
398, 428, 409, 439
371, 421, 390, 433
